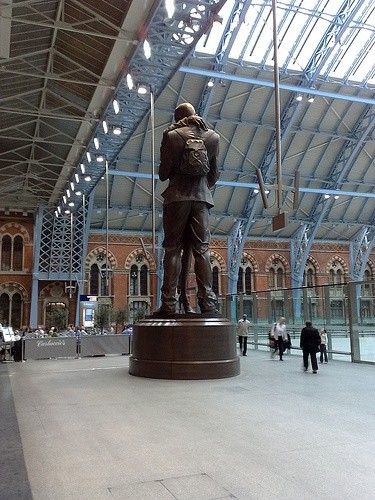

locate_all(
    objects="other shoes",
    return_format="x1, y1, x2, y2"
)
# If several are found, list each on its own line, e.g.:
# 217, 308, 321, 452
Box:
313, 370, 317, 374
279, 358, 284, 361
302, 368, 307, 371
271, 353, 274, 358
325, 362, 328, 364
318, 361, 322, 363
242, 354, 247, 356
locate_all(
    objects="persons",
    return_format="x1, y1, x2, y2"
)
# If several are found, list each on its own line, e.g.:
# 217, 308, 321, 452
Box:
300, 320, 321, 374
22, 326, 33, 337
152, 103, 220, 312
66, 324, 86, 336
320, 329, 329, 364
111, 324, 115, 332
49, 330, 57, 337
237, 314, 250, 356
36, 325, 44, 334
271, 317, 288, 361
11, 329, 22, 363
122, 324, 133, 333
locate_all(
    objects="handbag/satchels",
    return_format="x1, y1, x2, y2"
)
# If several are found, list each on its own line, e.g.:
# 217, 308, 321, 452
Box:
268, 323, 277, 341
